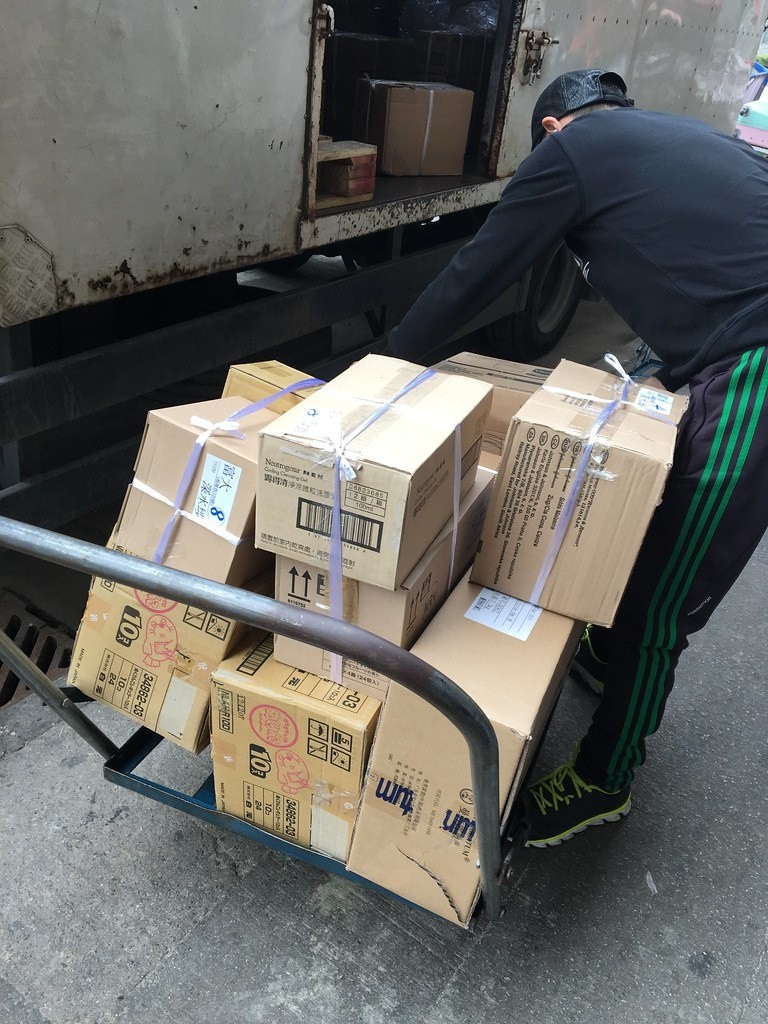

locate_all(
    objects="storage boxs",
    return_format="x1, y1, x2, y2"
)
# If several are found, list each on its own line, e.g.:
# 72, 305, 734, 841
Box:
326, 27, 492, 176
67, 352, 692, 931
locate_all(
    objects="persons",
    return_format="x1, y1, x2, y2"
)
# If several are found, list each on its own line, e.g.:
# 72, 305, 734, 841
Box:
378, 70, 768, 851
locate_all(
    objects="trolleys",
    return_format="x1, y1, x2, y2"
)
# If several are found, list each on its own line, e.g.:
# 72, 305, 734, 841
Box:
1, 517, 570, 938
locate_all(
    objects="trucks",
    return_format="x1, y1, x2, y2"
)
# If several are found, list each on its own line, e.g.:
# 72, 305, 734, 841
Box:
2, 1, 768, 705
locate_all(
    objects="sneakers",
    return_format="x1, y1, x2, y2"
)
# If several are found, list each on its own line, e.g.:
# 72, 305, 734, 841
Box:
506, 741, 634, 848
574, 622, 607, 693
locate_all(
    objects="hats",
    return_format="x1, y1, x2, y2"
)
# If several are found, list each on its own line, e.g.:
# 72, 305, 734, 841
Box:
531, 68, 634, 151
